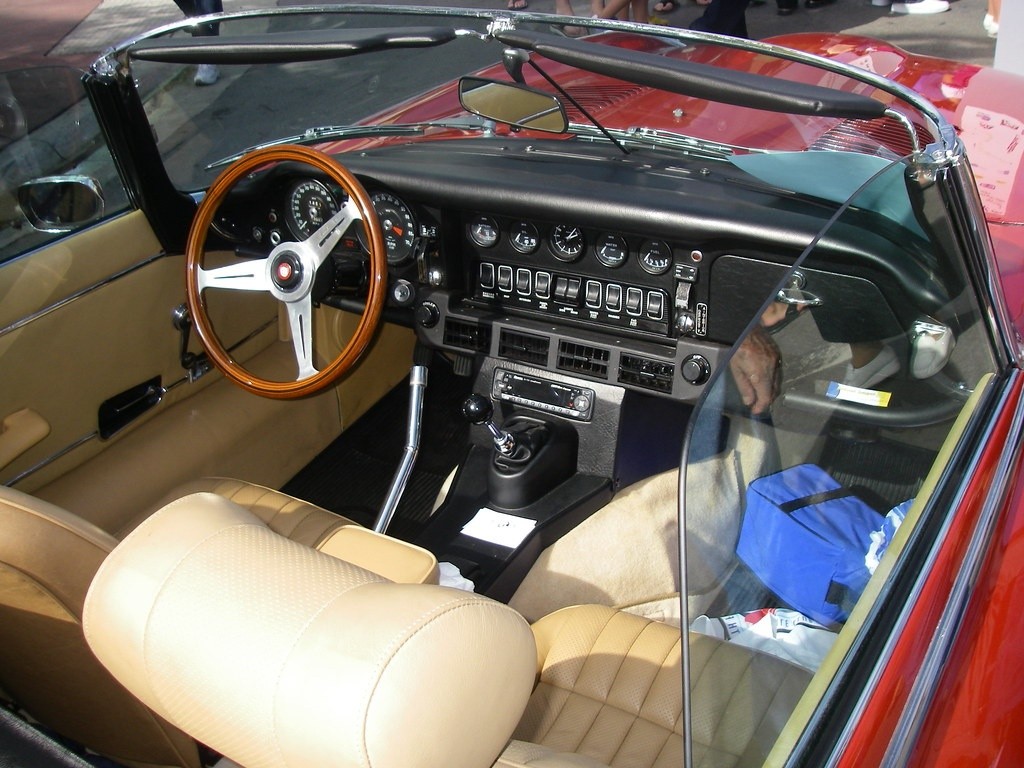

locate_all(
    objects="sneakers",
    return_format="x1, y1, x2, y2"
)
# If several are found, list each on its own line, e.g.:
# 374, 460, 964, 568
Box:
194, 63, 221, 86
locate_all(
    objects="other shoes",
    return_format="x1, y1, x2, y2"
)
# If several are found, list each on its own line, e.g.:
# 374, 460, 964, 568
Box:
988, 22, 998, 38
908, 327, 956, 379
777, 3, 798, 16
891, 1, 951, 14
836, 341, 901, 392
872, 1, 891, 7
983, 13, 993, 30
805, 0, 834, 11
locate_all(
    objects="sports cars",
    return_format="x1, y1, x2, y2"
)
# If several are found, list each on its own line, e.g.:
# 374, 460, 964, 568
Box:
1, 3, 1024, 768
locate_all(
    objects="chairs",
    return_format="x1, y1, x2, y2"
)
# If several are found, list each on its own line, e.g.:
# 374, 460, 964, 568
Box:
0, 477, 440, 768
81, 491, 816, 768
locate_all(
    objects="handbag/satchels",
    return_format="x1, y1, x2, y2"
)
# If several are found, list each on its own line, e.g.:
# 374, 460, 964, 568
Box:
735, 464, 892, 635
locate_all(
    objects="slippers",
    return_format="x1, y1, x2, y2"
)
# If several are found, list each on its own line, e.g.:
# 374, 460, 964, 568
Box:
509, 0, 528, 11
549, 21, 591, 40
763, 299, 813, 335
654, 0, 681, 15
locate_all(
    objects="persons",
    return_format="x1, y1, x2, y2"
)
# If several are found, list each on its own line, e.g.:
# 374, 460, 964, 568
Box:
679, 292, 806, 608
176, 0, 996, 85
